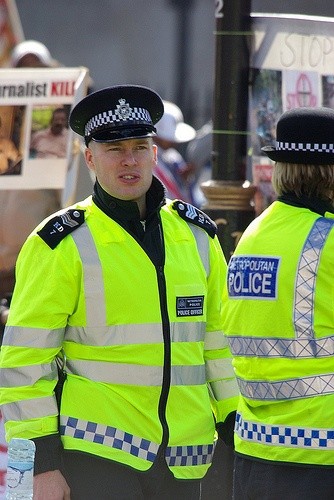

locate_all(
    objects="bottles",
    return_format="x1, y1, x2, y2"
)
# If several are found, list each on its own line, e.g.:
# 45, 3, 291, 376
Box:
6, 438, 37, 500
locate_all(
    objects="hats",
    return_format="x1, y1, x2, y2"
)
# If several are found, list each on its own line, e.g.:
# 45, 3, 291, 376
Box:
11, 38, 51, 69
68, 83, 165, 148
261, 106, 334, 165
155, 100, 196, 143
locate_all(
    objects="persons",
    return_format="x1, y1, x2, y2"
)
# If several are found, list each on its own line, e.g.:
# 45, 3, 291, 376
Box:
9, 39, 51, 69
0, 115, 22, 175
0, 84, 239, 500
152, 98, 198, 205
30, 108, 68, 158
220, 107, 334, 500
